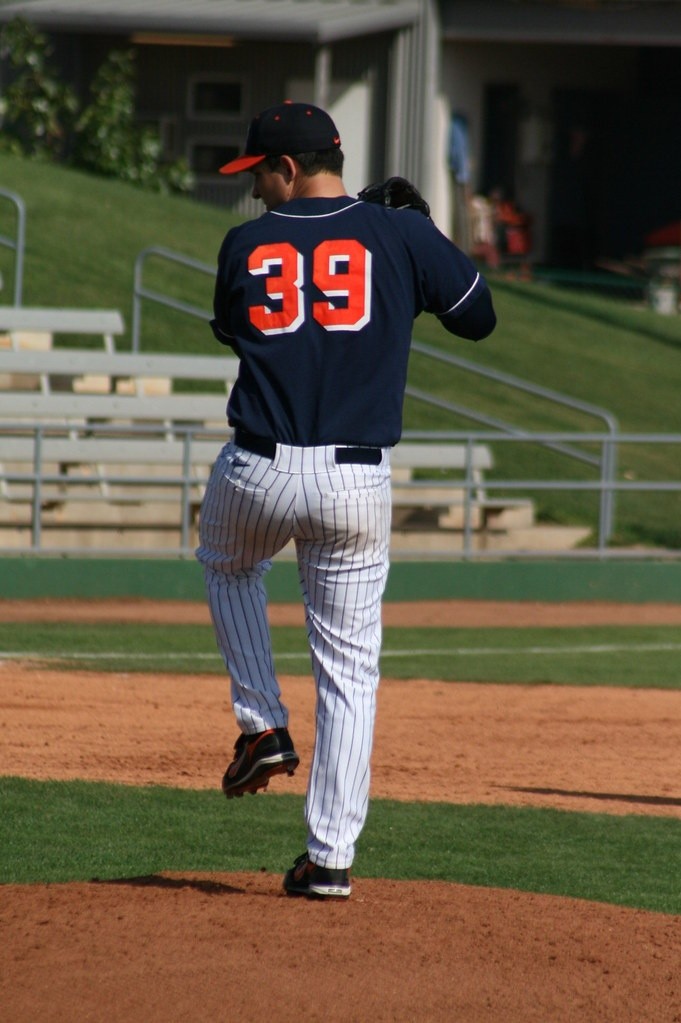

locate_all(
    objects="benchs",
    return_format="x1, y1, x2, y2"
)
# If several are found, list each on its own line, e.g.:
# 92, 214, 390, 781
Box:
0, 308, 534, 550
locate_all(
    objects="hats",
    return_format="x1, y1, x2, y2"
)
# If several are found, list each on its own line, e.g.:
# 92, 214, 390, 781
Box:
218, 100, 341, 174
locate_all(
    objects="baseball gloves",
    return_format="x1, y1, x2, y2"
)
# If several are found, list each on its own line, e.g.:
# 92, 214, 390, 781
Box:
357, 177, 431, 220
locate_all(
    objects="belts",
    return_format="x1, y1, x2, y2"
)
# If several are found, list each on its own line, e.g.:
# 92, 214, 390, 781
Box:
235, 429, 382, 465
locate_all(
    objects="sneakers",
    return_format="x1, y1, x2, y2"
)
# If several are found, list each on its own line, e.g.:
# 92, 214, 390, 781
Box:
223, 725, 299, 800
281, 850, 350, 898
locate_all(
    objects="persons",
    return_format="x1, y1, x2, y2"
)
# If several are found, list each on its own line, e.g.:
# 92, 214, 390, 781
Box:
374, 113, 473, 260
196, 103, 497, 898
